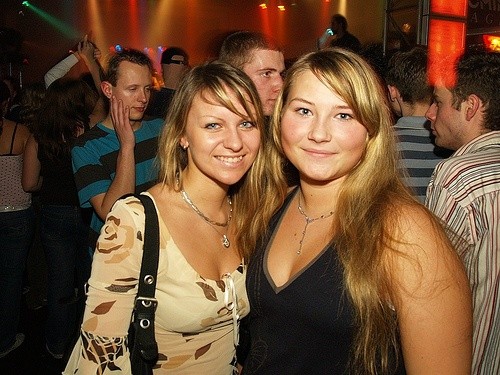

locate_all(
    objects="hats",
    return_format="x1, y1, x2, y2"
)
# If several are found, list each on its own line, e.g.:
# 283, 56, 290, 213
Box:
160, 47, 189, 65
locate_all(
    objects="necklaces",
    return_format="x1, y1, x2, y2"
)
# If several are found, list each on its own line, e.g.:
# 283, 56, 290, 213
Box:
295, 186, 338, 254
175, 171, 234, 248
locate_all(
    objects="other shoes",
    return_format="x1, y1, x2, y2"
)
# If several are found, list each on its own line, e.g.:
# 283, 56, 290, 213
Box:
41, 343, 64, 359
0, 332, 24, 359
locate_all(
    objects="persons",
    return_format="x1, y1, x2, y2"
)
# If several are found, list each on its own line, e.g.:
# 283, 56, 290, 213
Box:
386, 44, 456, 214
1, 31, 112, 358
423, 43, 500, 375
56, 63, 295, 374
220, 34, 287, 120
0, 79, 46, 358
234, 47, 473, 375
72, 48, 169, 265
330, 13, 364, 58
